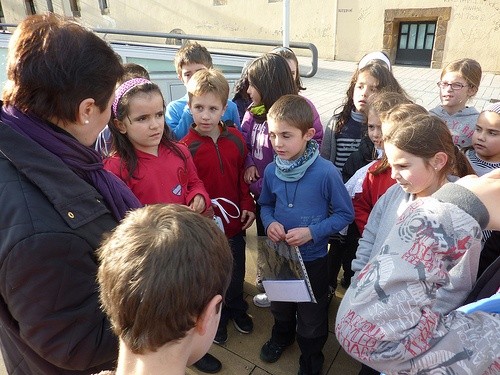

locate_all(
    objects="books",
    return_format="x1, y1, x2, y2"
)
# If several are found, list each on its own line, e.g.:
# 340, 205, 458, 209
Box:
261, 280, 312, 303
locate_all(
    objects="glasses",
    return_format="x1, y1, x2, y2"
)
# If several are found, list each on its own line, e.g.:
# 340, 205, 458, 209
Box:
437, 80, 471, 90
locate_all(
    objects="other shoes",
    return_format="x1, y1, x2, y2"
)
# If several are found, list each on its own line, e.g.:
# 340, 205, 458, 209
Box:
192, 353, 222, 374
253, 293, 271, 307
327, 285, 335, 306
256, 276, 265, 292
341, 278, 348, 289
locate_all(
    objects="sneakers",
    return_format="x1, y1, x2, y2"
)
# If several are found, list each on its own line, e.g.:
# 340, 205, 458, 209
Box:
213, 324, 228, 344
298, 355, 324, 375
231, 314, 253, 334
260, 336, 294, 363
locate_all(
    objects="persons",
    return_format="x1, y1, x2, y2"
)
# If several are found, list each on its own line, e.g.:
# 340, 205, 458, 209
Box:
336, 172, 499, 373
341, 92, 414, 180
93, 204, 234, 375
163, 44, 241, 140
320, 62, 408, 171
259, 94, 354, 375
178, 69, 256, 344
465, 104, 500, 177
102, 74, 221, 372
0, 12, 144, 374
351, 114, 492, 272
96, 63, 182, 158
423, 59, 482, 148
357, 51, 391, 71
240, 53, 323, 308
232, 48, 308, 124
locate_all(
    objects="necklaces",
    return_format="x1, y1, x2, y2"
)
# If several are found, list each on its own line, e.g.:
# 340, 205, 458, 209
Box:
284, 181, 298, 206
